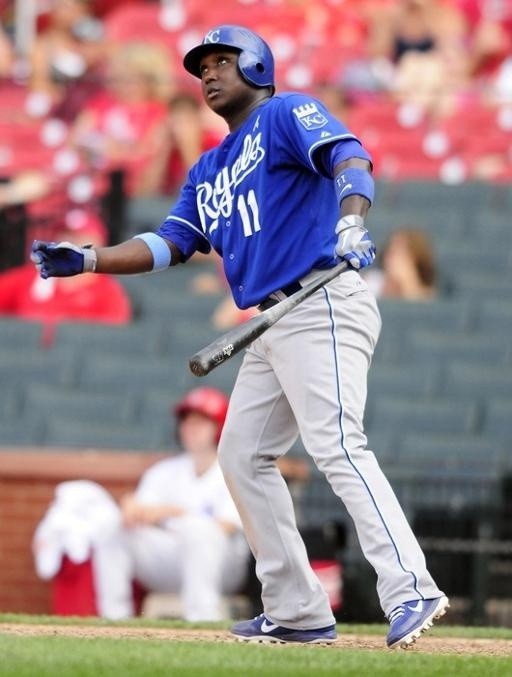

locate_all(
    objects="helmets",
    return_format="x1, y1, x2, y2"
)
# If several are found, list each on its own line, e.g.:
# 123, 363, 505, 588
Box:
175, 385, 228, 444
183, 24, 275, 96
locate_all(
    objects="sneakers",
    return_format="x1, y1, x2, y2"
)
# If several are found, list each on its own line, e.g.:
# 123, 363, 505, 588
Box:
386, 595, 450, 649
229, 612, 338, 645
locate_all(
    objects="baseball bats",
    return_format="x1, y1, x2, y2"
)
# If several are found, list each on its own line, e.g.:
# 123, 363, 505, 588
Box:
189, 243, 375, 378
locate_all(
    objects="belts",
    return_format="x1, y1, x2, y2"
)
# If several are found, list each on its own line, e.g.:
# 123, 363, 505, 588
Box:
258, 280, 303, 312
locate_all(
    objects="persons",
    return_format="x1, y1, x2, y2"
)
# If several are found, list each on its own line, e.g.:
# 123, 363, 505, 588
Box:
0, 0, 511, 328
92, 387, 252, 624
31, 26, 452, 650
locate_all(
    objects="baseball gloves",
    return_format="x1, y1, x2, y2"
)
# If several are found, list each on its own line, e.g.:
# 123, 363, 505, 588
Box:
385, 225, 432, 303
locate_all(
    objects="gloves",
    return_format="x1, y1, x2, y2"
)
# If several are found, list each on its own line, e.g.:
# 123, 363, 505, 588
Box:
335, 215, 377, 273
30, 240, 97, 280
